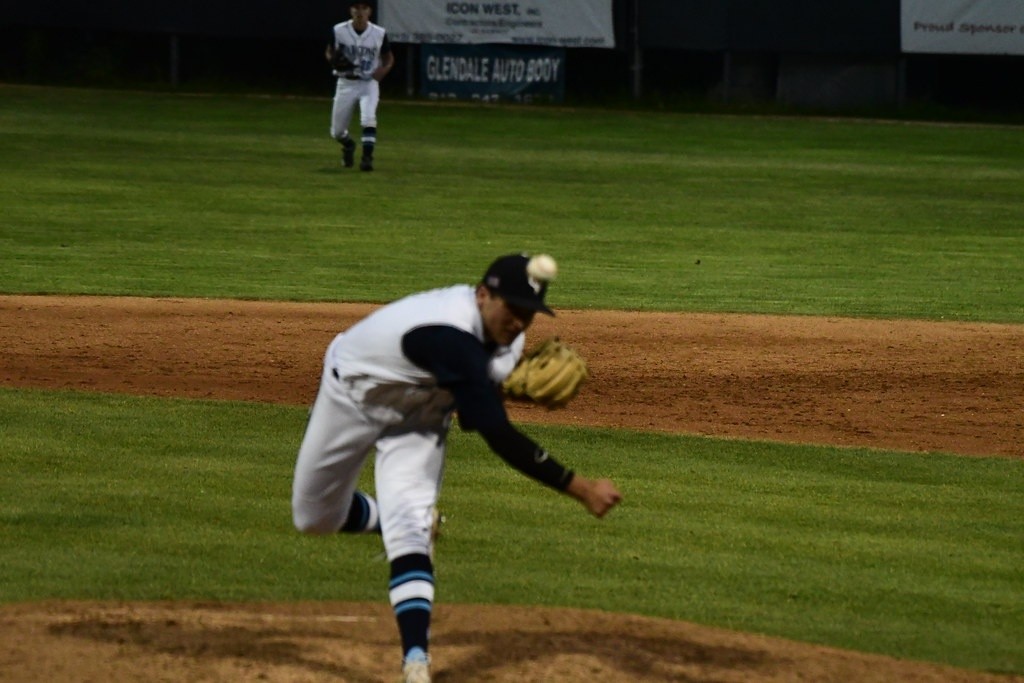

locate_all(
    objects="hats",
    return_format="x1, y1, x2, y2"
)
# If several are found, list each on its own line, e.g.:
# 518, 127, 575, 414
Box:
482, 253, 557, 318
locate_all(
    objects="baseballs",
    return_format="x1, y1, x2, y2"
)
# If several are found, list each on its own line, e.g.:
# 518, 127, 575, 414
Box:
526, 252, 557, 283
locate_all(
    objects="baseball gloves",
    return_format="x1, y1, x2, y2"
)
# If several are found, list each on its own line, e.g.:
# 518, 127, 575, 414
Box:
499, 336, 594, 409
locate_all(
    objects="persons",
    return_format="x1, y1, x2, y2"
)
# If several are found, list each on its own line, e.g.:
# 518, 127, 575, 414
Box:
291, 250, 627, 683
321, 0, 396, 174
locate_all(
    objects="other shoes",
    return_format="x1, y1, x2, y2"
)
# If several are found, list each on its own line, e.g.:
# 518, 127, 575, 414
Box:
401, 662, 432, 683
342, 139, 374, 172
